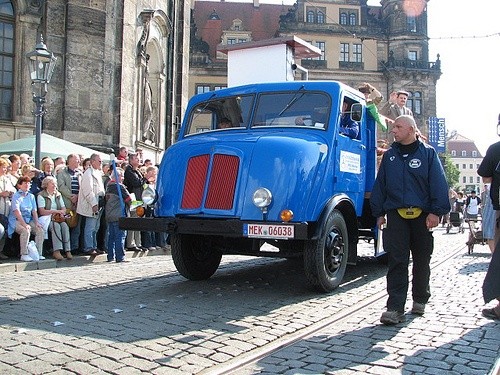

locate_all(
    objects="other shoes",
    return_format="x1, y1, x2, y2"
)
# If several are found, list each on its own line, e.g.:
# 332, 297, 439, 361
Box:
0, 253, 8, 259
148, 246, 155, 250
39, 255, 45, 260
482, 302, 500, 319
66, 251, 72, 259
95, 249, 105, 254
139, 246, 148, 251
85, 250, 99, 255
109, 259, 114, 262
116, 259, 129, 263
20, 254, 33, 261
53, 250, 64, 260
155, 246, 161, 249
127, 246, 142, 251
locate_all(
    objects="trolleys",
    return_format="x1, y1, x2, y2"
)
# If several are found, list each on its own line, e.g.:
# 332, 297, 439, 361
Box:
445, 212, 466, 234
467, 218, 487, 254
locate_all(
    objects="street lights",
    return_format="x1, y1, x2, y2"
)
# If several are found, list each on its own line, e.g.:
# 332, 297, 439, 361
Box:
25, 32, 57, 165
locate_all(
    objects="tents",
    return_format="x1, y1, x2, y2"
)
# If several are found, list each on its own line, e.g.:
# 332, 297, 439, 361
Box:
0, 133, 116, 164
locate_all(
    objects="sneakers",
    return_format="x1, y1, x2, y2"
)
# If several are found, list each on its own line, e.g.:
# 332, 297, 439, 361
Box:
381, 310, 406, 324
413, 301, 424, 314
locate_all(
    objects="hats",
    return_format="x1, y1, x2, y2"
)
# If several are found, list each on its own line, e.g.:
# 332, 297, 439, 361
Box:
397, 90, 409, 97
358, 86, 370, 93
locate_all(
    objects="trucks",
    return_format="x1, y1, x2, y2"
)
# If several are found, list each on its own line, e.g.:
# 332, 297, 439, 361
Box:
115, 80, 398, 292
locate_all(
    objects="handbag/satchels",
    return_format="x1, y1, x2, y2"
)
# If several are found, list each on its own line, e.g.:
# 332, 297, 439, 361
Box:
26, 240, 39, 262
53, 208, 78, 228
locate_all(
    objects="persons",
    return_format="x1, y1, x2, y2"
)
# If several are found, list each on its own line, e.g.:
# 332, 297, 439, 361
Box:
218, 112, 233, 129
359, 82, 383, 104
295, 98, 359, 139
379, 90, 427, 140
370, 115, 451, 324
478, 116, 500, 319
0, 147, 173, 263
444, 187, 481, 227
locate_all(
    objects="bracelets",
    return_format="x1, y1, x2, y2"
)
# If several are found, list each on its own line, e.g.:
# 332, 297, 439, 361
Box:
372, 89, 374, 91
